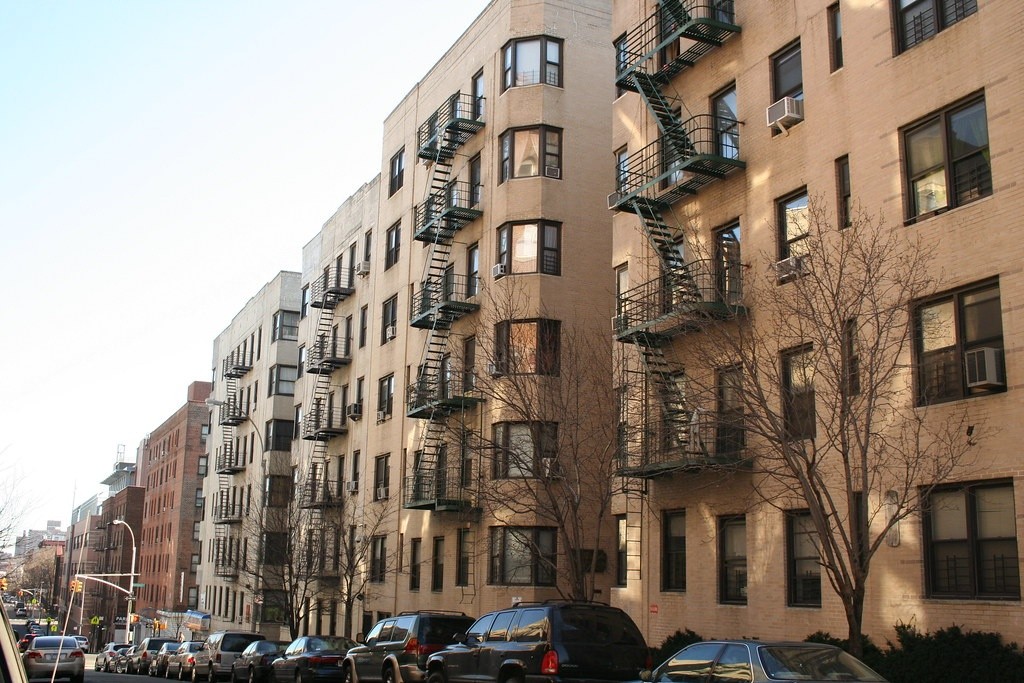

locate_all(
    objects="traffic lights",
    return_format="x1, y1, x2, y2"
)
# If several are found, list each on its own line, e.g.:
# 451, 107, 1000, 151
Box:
133, 615, 138, 623
154, 621, 160, 631
0, 578, 7, 592
69, 580, 76, 592
76, 580, 82, 592
18, 591, 23, 596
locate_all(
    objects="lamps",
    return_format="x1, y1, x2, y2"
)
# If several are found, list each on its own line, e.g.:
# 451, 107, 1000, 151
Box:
610, 313, 629, 332
605, 191, 626, 213
357, 592, 365, 602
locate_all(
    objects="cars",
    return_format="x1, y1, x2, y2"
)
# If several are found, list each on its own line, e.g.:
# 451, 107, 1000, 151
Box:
70, 636, 90, 654
267, 635, 360, 683
21, 635, 86, 683
164, 640, 206, 680
229, 639, 293, 683
16, 608, 27, 617
2, 592, 24, 611
25, 618, 43, 634
106, 645, 139, 675
637, 639, 892, 683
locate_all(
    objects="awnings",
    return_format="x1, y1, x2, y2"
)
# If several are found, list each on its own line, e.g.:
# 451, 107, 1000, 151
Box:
137, 608, 161, 625
184, 611, 211, 630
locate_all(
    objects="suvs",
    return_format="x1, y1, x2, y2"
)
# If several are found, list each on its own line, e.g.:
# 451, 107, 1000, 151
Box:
131, 637, 178, 675
424, 598, 654, 683
95, 642, 128, 672
342, 609, 477, 683
190, 630, 266, 683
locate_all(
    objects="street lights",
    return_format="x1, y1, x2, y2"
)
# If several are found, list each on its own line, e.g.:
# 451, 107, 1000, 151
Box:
205, 398, 267, 633
113, 519, 137, 645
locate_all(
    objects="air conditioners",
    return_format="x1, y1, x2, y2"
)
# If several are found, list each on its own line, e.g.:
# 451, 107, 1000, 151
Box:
376, 411, 386, 422
355, 261, 369, 275
488, 361, 505, 376
492, 264, 506, 278
775, 256, 802, 282
346, 481, 358, 492
345, 403, 362, 418
386, 327, 396, 340
539, 458, 561, 479
766, 96, 804, 129
376, 487, 389, 500
546, 166, 561, 179
964, 348, 1004, 390
